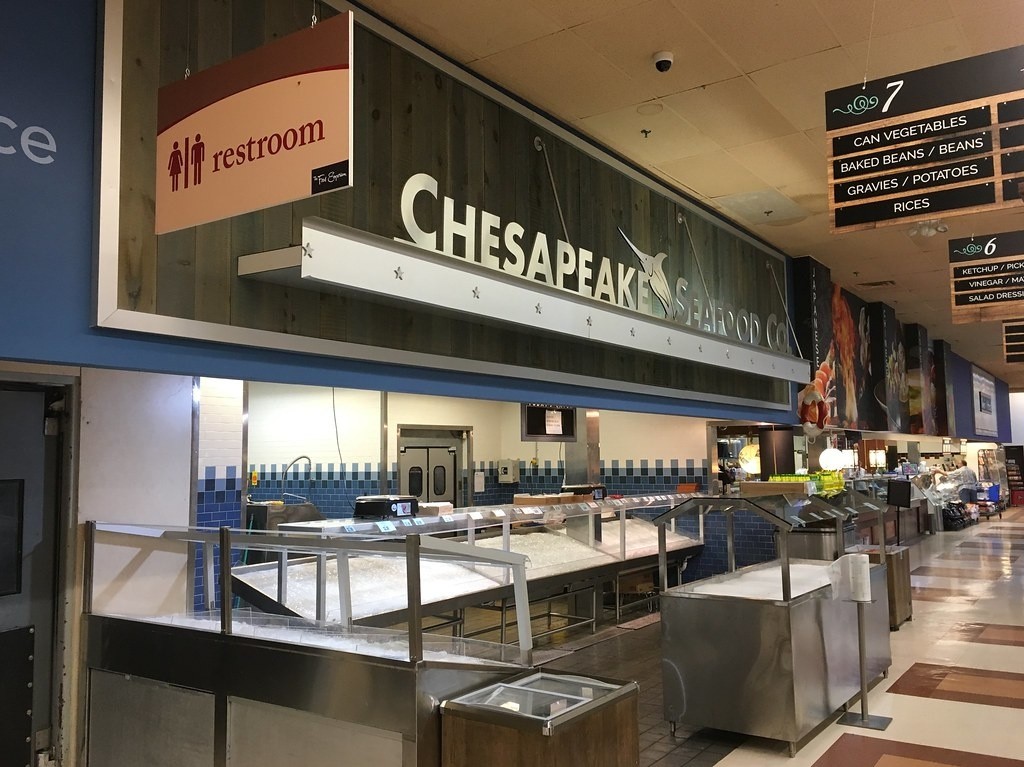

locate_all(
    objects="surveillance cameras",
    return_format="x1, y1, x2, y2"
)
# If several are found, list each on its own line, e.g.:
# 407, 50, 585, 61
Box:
653, 51, 674, 72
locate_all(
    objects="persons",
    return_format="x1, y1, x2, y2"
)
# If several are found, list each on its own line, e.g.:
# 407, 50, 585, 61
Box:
894, 458, 906, 473
853, 462, 868, 495
935, 461, 978, 505
